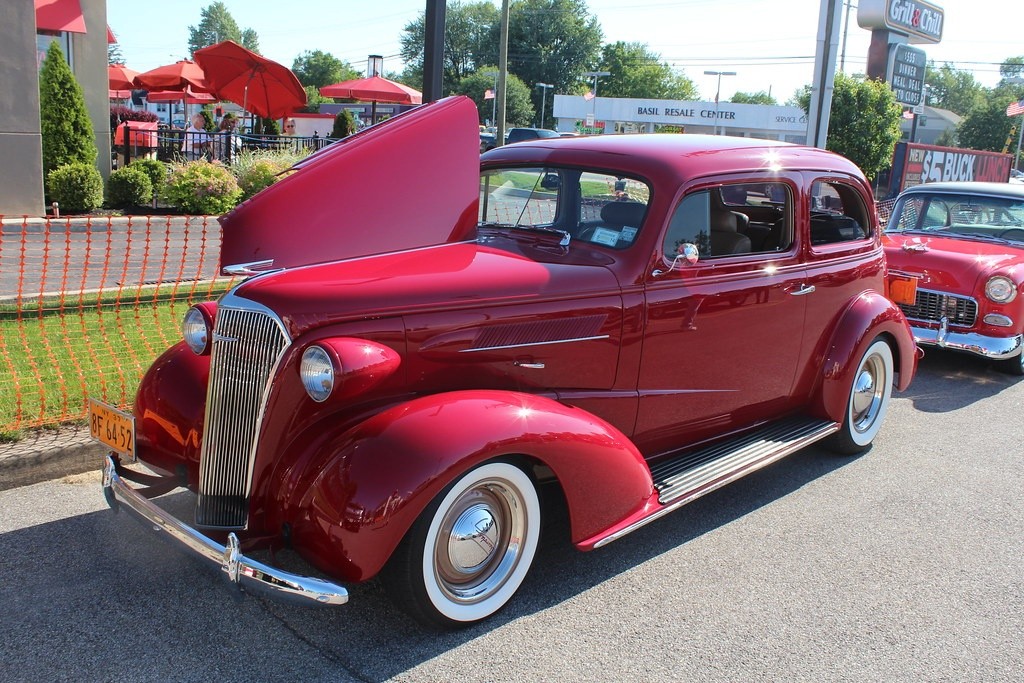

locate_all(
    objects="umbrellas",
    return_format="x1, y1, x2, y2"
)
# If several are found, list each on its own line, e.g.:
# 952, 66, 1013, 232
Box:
133, 57, 207, 123
194, 39, 307, 126
108, 63, 141, 120
147, 84, 215, 114
319, 76, 422, 125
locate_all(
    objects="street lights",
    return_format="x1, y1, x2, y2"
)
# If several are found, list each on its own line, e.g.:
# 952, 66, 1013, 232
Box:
705, 70, 736, 135
583, 72, 610, 134
485, 71, 509, 136
536, 82, 554, 129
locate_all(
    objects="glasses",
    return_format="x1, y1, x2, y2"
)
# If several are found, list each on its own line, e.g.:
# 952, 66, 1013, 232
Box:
286, 125, 294, 128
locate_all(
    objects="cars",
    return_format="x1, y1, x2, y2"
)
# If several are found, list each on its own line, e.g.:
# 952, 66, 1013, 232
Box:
479, 125, 498, 142
875, 196, 1021, 227
881, 181, 1024, 378
484, 128, 579, 152
1010, 168, 1024, 182
166, 119, 192, 129
88, 95, 924, 631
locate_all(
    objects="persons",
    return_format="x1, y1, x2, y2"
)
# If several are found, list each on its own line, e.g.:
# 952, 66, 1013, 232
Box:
211, 120, 242, 163
282, 118, 304, 149
181, 113, 211, 161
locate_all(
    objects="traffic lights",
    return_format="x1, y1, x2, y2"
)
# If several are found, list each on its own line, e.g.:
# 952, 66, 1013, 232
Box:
217, 109, 221, 117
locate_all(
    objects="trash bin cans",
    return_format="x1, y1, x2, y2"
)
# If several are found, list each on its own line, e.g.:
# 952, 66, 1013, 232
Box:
114, 121, 158, 170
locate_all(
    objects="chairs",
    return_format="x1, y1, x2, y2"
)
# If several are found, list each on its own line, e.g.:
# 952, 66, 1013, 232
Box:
578, 201, 646, 250
710, 208, 752, 254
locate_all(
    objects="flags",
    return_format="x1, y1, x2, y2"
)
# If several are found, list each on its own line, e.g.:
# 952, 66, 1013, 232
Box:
902, 110, 914, 118
1006, 100, 1024, 116
484, 90, 495, 99
584, 89, 596, 101
